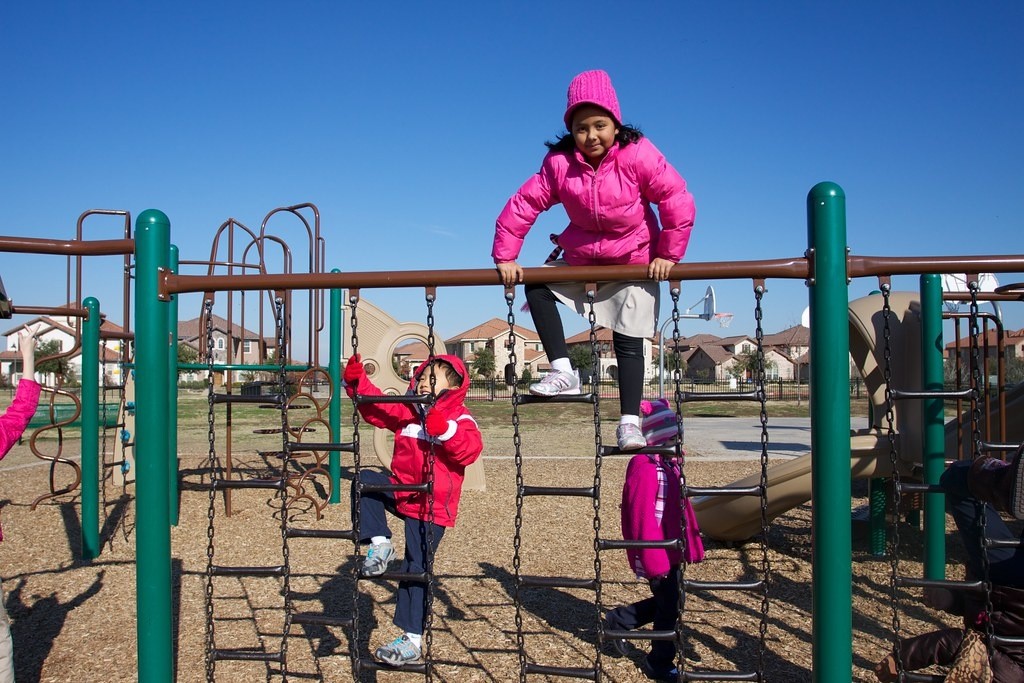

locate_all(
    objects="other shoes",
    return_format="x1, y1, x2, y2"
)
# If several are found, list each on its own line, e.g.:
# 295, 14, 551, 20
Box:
644, 654, 689, 683
606, 611, 629, 656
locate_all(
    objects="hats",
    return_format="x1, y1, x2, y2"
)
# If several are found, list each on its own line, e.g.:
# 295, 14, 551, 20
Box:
564, 70, 623, 132
641, 398, 678, 461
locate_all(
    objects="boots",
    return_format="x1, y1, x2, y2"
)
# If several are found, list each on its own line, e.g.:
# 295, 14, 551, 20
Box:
968, 441, 1024, 521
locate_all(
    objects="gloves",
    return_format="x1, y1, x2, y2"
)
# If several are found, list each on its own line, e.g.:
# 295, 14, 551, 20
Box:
343, 353, 363, 387
425, 407, 448, 436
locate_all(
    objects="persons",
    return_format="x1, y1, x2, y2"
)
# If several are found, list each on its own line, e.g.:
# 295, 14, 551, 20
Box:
345, 355, 483, 665
492, 69, 696, 449
875, 440, 1024, 683
0, 324, 42, 683
606, 398, 705, 683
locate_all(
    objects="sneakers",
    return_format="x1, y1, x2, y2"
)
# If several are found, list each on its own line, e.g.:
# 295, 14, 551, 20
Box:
529, 369, 581, 396
375, 634, 422, 666
616, 423, 647, 451
361, 539, 396, 577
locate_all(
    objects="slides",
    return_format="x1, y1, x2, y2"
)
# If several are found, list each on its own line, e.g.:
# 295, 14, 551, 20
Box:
690, 290, 924, 544
